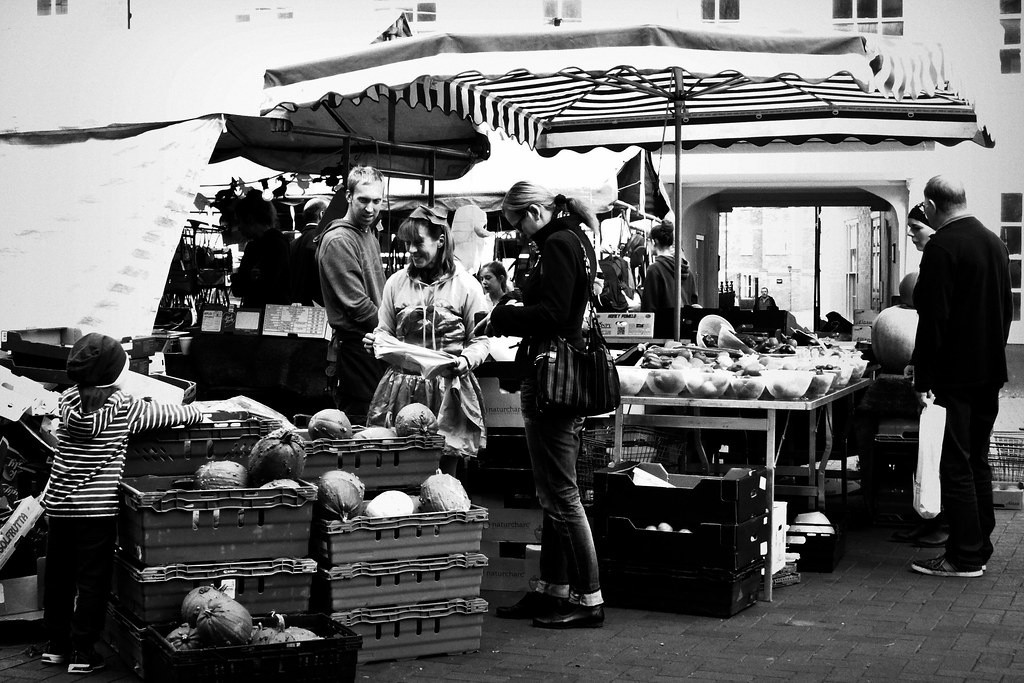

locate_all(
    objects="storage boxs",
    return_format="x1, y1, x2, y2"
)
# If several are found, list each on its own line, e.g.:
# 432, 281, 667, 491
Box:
120, 423, 495, 683
472, 375, 527, 432
593, 459, 771, 618
480, 557, 553, 594
487, 506, 546, 546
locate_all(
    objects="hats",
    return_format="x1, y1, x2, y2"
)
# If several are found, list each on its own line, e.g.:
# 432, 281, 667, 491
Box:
907, 201, 941, 230
408, 204, 449, 226
65, 332, 126, 387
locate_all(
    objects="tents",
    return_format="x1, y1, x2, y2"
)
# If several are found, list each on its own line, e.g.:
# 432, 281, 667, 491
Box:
0, 11, 496, 356
189, 141, 677, 238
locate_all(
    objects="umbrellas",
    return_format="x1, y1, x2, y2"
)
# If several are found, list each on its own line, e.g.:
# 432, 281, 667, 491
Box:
261, 21, 997, 339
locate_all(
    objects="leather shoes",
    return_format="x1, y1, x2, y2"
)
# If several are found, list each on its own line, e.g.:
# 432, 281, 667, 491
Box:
494, 592, 574, 620
890, 516, 950, 549
530, 606, 605, 629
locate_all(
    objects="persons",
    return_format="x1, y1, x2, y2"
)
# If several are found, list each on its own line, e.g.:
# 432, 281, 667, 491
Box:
313, 165, 389, 427
892, 201, 952, 548
288, 195, 330, 302
912, 178, 1014, 578
41, 332, 203, 674
229, 198, 295, 313
641, 220, 696, 337
493, 181, 623, 629
753, 287, 776, 310
470, 262, 524, 337
361, 205, 488, 482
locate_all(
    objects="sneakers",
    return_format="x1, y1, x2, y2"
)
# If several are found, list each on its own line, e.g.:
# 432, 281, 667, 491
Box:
907, 553, 989, 578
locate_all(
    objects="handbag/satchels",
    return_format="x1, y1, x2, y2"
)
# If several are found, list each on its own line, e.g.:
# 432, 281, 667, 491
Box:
912, 396, 946, 520
535, 319, 622, 416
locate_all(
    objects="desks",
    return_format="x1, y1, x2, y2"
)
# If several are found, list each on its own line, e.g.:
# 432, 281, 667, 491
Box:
604, 376, 880, 599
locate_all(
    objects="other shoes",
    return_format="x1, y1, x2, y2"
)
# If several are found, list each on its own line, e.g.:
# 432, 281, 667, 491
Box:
38, 640, 106, 674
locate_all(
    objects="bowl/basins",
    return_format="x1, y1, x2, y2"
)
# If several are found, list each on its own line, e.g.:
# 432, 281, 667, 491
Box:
615, 344, 870, 397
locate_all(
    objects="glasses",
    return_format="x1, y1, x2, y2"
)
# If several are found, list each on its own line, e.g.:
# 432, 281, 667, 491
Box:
514, 214, 527, 232
